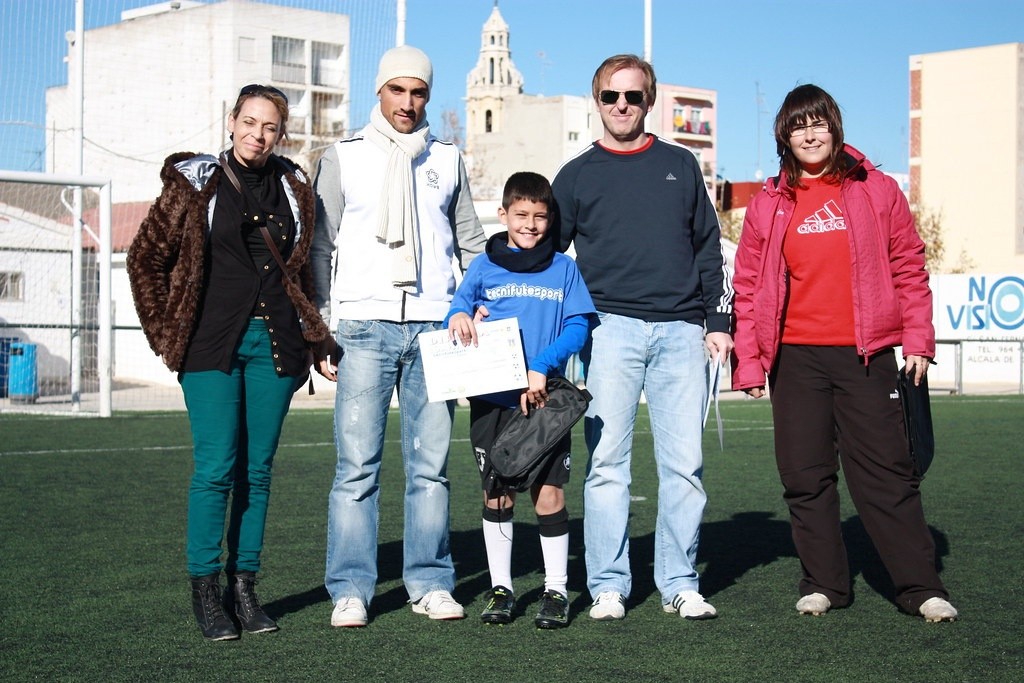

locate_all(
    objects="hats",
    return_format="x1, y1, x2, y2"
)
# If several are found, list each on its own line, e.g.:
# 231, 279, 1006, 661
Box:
375, 44, 433, 102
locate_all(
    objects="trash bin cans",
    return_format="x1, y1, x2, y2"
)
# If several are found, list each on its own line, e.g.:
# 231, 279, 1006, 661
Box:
0, 337, 19, 397
8, 343, 39, 404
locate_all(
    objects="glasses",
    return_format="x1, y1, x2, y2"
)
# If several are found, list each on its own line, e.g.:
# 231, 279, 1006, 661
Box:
596, 90, 648, 105
788, 120, 833, 137
238, 83, 288, 99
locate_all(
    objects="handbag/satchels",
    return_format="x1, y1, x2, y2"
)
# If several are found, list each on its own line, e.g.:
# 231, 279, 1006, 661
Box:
488, 378, 591, 494
897, 363, 936, 477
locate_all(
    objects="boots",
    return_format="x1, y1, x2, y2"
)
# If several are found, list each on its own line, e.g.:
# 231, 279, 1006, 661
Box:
191, 572, 279, 641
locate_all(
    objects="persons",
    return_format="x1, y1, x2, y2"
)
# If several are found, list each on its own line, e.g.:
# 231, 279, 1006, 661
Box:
444, 172, 600, 631
550, 56, 735, 622
729, 84, 961, 621
127, 84, 345, 641
290, 45, 489, 626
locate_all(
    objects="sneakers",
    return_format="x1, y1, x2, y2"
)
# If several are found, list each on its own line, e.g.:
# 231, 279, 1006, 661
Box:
664, 590, 718, 619
481, 585, 518, 625
535, 590, 572, 629
411, 588, 467, 620
588, 590, 629, 619
796, 592, 832, 616
331, 597, 371, 627
919, 597, 958, 624
